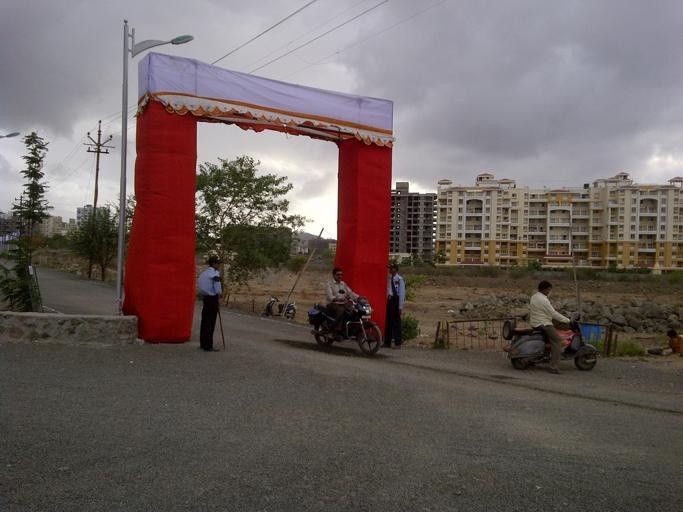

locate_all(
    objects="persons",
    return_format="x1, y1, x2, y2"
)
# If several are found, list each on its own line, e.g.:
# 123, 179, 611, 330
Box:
528, 280, 576, 374
196, 256, 224, 352
665, 329, 683, 358
379, 263, 405, 349
324, 268, 367, 342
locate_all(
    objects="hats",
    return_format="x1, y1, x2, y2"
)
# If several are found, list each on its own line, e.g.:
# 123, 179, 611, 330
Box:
387, 264, 399, 268
208, 256, 221, 264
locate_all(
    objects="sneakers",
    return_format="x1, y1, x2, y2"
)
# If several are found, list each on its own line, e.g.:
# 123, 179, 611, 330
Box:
550, 369, 563, 373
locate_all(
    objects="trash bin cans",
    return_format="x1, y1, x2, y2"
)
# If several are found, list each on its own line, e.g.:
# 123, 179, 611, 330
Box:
577, 322, 605, 342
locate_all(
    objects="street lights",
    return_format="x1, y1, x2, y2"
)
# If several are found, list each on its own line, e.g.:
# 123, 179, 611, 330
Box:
115, 18, 194, 318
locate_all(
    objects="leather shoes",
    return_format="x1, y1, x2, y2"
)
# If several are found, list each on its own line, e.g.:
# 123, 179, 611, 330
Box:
381, 344, 401, 349
200, 345, 220, 352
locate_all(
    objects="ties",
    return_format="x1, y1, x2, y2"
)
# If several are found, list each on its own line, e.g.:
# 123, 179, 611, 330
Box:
391, 276, 397, 296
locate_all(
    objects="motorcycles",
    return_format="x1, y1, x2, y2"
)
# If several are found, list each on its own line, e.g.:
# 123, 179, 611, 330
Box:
262, 295, 297, 319
307, 289, 382, 355
501, 306, 597, 371
